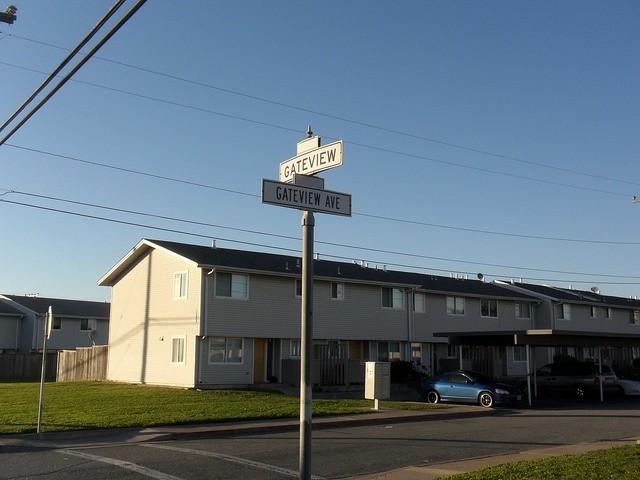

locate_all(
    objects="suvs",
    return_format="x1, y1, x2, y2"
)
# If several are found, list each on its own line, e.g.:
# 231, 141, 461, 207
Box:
519, 361, 618, 404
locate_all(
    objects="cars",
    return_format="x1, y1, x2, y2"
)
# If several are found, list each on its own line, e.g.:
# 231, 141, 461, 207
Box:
427, 370, 524, 407
616, 371, 640, 401
